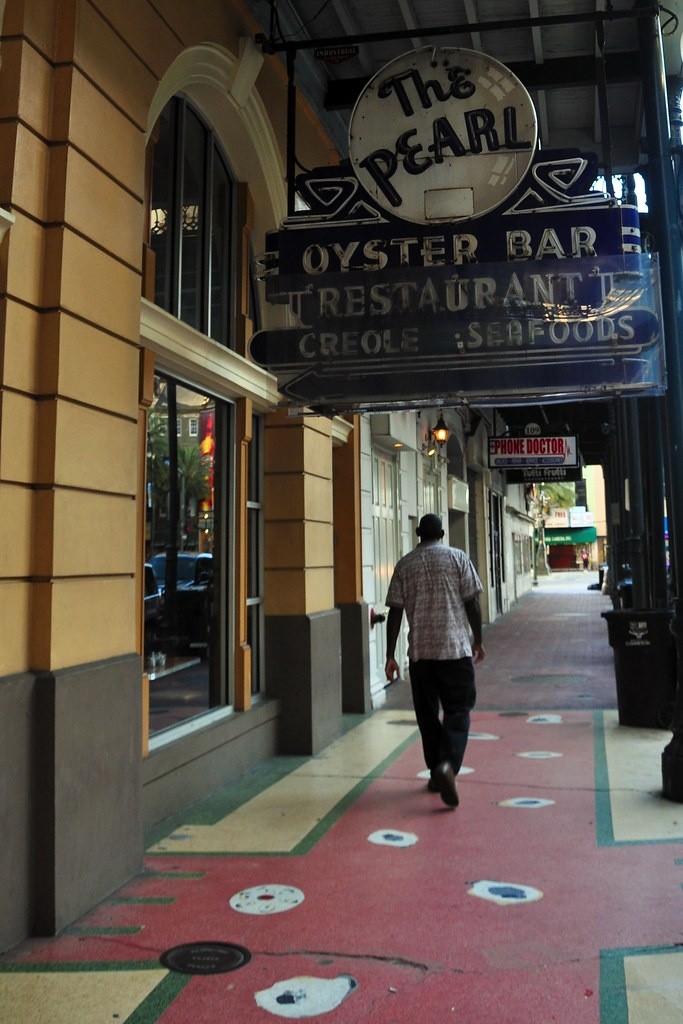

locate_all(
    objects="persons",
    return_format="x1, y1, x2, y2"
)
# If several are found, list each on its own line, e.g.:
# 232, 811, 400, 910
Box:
581, 548, 589, 572
385, 513, 485, 808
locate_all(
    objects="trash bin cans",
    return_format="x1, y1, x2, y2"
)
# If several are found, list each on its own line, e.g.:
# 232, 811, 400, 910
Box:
599, 562, 608, 588
617, 580, 634, 609
600, 607, 677, 732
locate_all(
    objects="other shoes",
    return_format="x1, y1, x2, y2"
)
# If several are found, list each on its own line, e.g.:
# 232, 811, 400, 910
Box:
428, 761, 459, 806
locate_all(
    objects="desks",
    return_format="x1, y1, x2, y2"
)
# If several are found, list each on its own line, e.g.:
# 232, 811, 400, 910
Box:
144, 656, 201, 681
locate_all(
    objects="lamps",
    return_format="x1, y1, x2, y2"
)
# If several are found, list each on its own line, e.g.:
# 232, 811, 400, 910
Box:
428, 412, 448, 448
